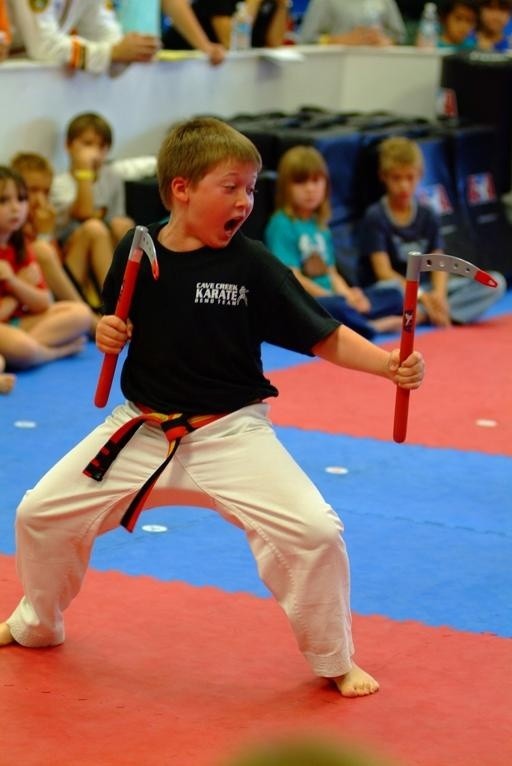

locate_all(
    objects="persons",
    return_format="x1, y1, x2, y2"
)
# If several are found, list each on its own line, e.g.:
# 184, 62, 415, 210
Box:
437, 0, 512, 53
270, 147, 421, 338
364, 137, 506, 327
0, 108, 133, 395
161, 1, 407, 64
0, 1, 160, 79
1, 115, 381, 698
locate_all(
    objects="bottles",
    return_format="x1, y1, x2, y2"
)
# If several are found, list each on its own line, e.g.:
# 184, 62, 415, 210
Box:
231, 1, 255, 51
416, 2, 445, 49
